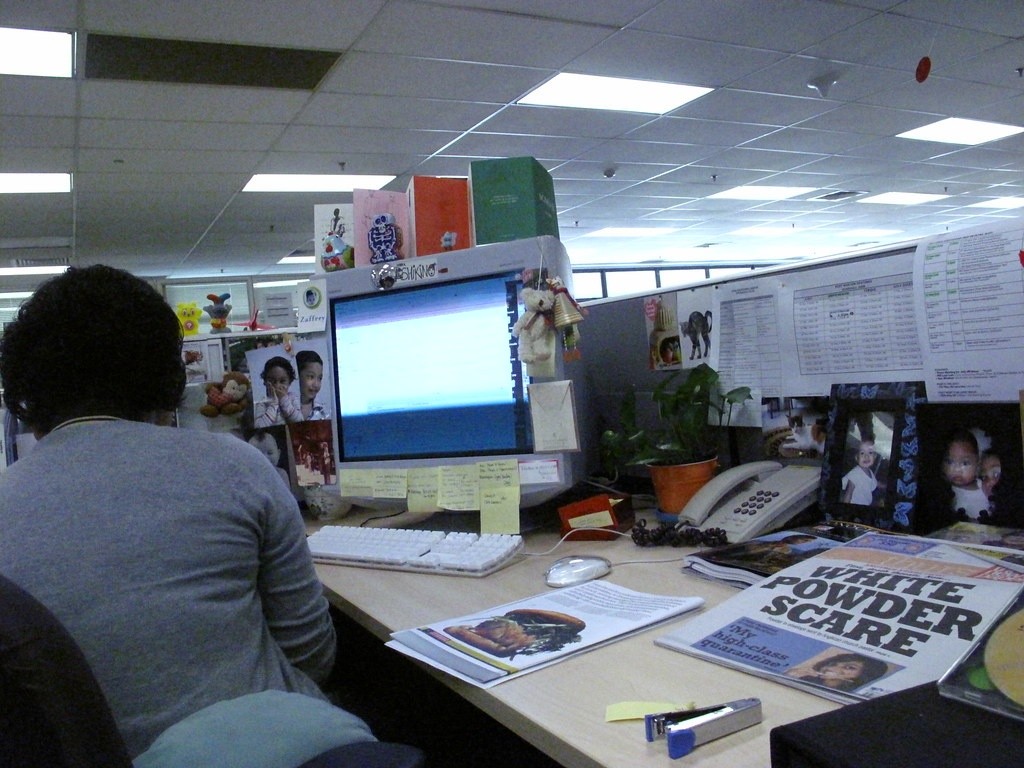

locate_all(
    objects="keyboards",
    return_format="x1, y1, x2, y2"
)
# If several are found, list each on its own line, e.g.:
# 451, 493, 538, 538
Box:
306, 524, 525, 578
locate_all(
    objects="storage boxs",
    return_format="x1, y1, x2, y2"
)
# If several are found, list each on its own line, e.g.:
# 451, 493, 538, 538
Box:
405, 173, 472, 259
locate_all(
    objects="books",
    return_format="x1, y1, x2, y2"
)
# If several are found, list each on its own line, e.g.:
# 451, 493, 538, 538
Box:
653, 532, 1024, 706
390, 580, 705, 683
681, 520, 909, 590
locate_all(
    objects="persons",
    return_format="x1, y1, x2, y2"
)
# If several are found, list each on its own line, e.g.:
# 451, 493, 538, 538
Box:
0, 264, 337, 759
249, 428, 290, 489
253, 357, 302, 428
942, 432, 1001, 518
784, 653, 888, 693
306, 290, 317, 305
841, 436, 877, 505
296, 350, 331, 421
760, 535, 817, 554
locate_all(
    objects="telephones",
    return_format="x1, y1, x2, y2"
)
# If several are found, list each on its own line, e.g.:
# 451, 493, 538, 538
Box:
675, 460, 822, 545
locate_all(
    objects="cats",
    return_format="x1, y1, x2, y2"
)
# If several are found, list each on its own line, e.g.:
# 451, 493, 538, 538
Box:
782, 411, 826, 455
679, 309, 714, 361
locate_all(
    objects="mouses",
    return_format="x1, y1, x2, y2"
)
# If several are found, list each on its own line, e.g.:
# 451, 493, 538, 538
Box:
543, 554, 612, 588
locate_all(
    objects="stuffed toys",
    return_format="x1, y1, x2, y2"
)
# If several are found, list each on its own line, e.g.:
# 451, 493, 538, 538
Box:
199, 371, 251, 419
512, 288, 555, 364
175, 302, 203, 336
203, 293, 233, 334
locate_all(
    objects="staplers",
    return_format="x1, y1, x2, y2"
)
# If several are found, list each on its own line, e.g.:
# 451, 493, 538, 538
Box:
645, 698, 763, 760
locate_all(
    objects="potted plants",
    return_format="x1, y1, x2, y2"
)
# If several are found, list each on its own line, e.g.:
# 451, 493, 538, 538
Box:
579, 362, 754, 517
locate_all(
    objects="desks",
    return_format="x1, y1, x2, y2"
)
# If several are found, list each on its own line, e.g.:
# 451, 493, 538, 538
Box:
291, 503, 843, 768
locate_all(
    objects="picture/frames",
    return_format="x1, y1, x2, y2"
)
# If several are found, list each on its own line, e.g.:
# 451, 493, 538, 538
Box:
824, 395, 909, 522
913, 401, 1024, 533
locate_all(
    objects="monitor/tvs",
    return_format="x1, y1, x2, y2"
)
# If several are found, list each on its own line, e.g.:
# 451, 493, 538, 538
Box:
311, 234, 587, 535
